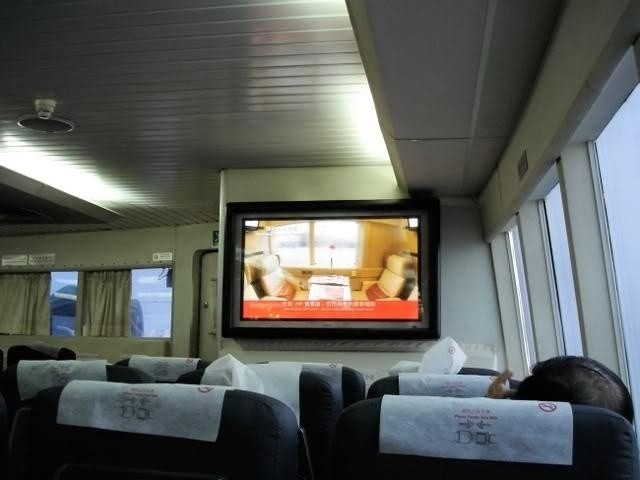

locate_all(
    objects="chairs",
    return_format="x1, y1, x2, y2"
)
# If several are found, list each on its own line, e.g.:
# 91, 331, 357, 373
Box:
329, 393, 639, 479
114, 355, 211, 384
190, 366, 343, 478
6, 379, 315, 477
256, 360, 366, 404
387, 364, 501, 377
7, 346, 77, 362
368, 375, 523, 403
2, 359, 155, 429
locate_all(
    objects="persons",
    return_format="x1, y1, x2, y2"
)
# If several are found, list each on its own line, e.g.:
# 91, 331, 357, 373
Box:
485, 353, 634, 422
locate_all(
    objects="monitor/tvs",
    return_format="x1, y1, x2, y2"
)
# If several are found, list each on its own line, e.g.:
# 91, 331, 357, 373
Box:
223, 199, 441, 339
